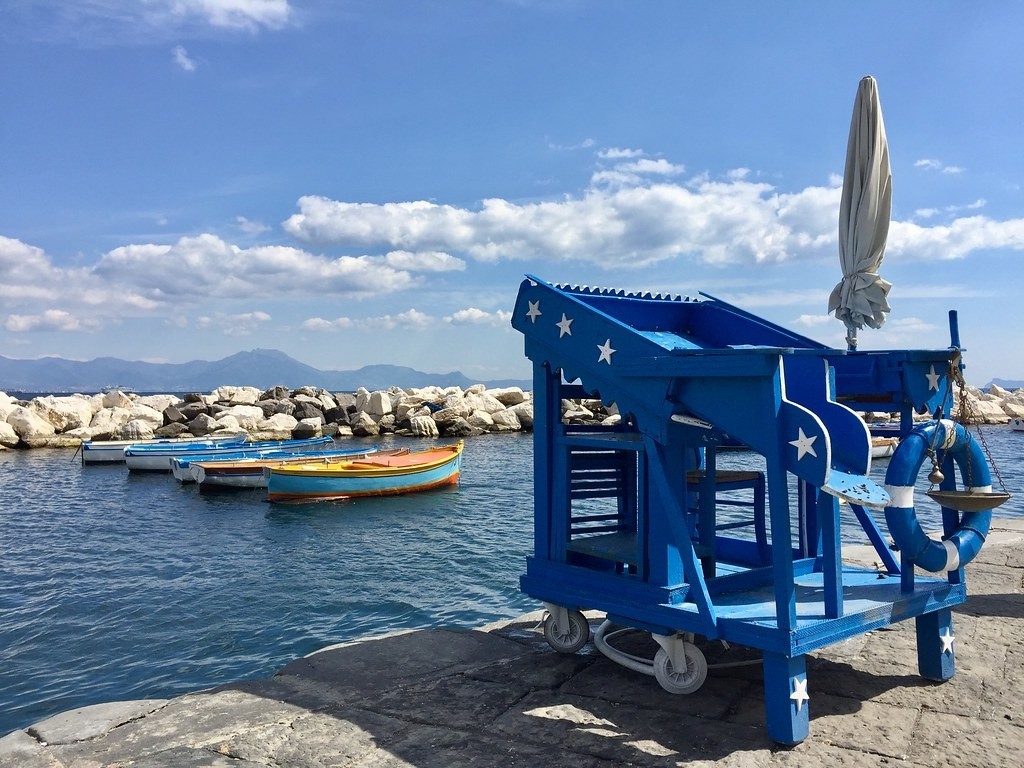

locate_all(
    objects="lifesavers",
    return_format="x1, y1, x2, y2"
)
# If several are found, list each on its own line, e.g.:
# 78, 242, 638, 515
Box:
881, 415, 998, 571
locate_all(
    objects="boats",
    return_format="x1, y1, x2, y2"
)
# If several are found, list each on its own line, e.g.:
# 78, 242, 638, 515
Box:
124, 434, 332, 470
168, 445, 379, 482
870, 437, 903, 458
263, 438, 466, 502
188, 447, 410, 487
81, 435, 247, 462
1008, 419, 1024, 431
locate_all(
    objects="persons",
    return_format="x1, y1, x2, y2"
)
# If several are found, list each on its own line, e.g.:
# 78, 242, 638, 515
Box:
594, 405, 608, 415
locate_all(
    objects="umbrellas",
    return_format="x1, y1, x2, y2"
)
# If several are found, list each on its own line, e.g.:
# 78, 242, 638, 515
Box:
827, 75, 892, 347
